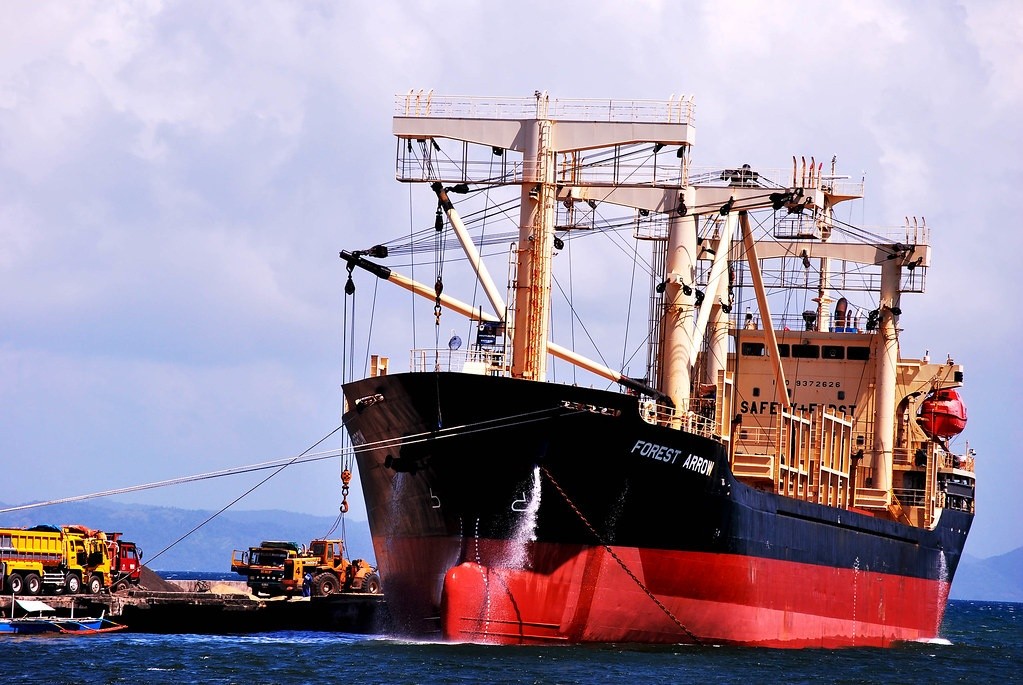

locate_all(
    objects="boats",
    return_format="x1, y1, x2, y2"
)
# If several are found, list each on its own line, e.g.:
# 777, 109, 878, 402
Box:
12, 610, 106, 636
0, 601, 56, 634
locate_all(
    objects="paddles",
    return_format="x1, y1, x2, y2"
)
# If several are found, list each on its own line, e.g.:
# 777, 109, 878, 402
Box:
44, 620, 65, 632
97, 618, 128, 628
68, 621, 101, 633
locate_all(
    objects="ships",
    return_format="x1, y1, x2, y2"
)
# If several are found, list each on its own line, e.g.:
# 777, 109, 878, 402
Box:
341, 83, 983, 652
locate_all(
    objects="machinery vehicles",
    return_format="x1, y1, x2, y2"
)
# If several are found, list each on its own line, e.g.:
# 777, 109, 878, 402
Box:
230, 541, 312, 597
0, 525, 113, 597
282, 539, 380, 597
106, 531, 143, 594
